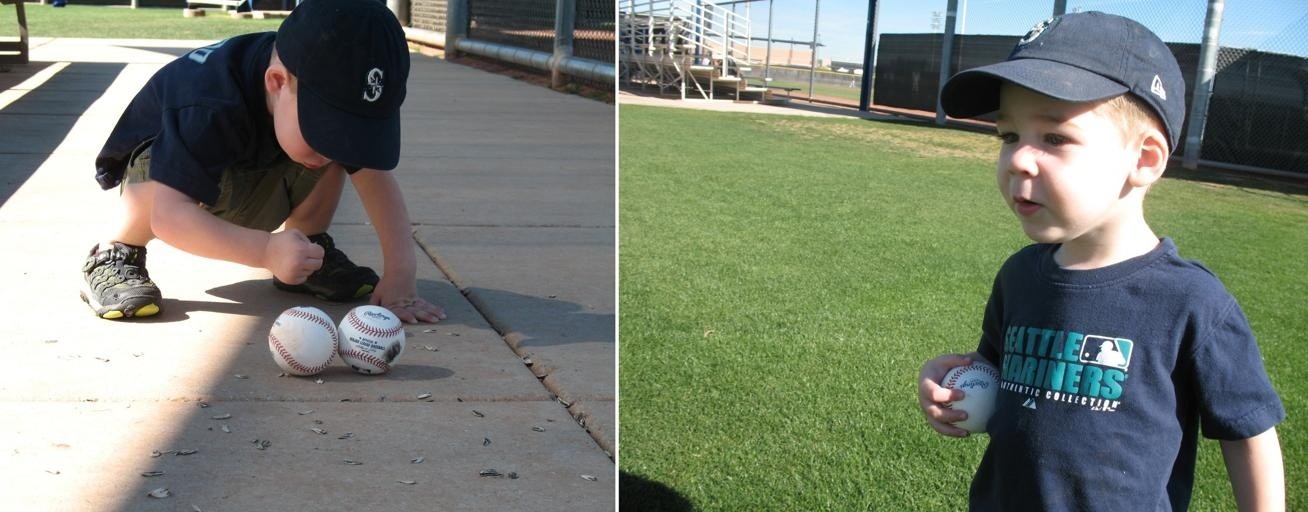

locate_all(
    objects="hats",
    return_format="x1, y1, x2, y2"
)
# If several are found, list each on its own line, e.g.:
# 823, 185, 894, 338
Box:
940, 10, 1188, 155
274, 1, 410, 172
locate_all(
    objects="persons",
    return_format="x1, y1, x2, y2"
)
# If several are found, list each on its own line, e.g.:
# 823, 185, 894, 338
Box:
920, 11, 1284, 512
79, 1, 448, 324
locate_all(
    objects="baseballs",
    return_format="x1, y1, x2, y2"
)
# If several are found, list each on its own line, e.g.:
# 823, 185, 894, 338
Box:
269, 305, 340, 379
941, 360, 1002, 433
339, 304, 405, 376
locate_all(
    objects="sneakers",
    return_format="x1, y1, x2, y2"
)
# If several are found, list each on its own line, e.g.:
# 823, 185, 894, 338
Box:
272, 230, 378, 302
77, 239, 162, 320
